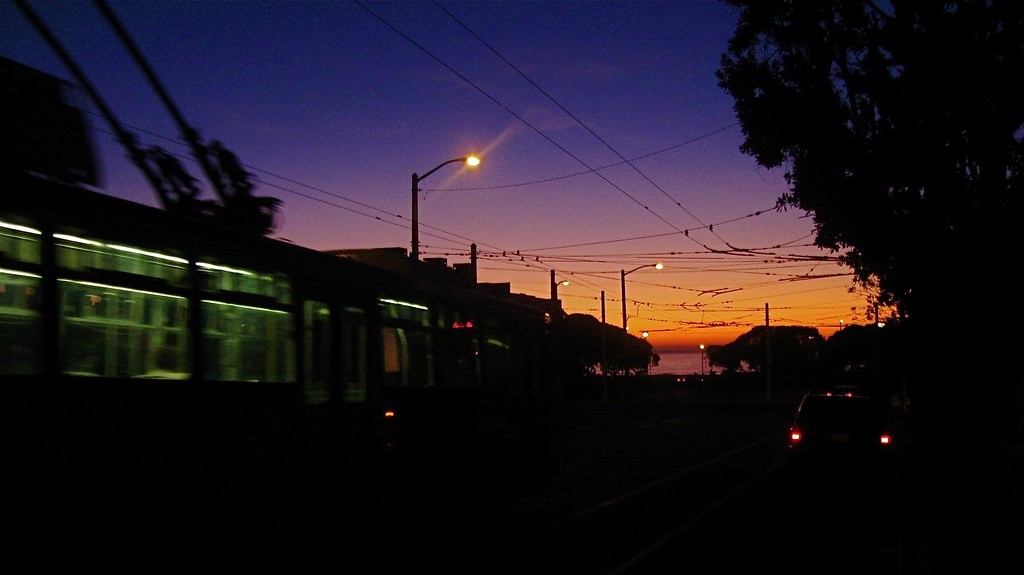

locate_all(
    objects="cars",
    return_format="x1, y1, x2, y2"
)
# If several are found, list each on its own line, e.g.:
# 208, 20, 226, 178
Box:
785, 385, 908, 465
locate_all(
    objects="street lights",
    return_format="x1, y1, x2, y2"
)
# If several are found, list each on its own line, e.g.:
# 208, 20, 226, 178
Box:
621, 262, 665, 392
699, 344, 705, 376
408, 153, 484, 260
839, 319, 844, 331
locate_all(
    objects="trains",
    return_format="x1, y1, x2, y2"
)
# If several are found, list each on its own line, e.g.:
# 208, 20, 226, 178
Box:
0, 154, 561, 413
